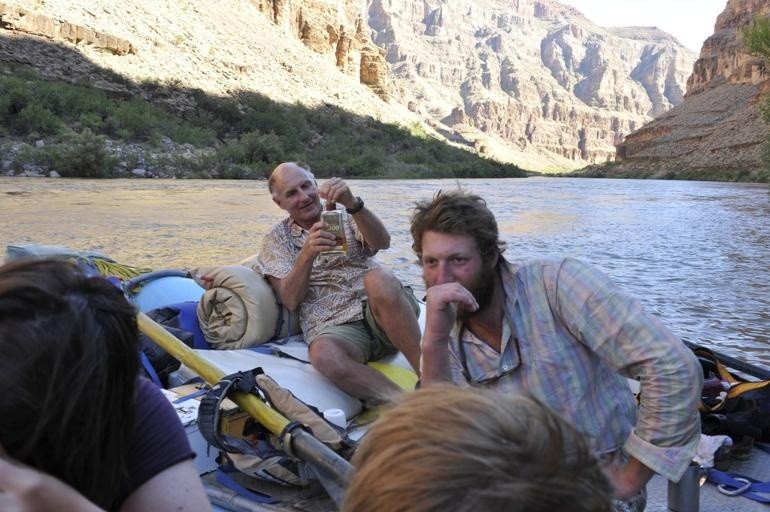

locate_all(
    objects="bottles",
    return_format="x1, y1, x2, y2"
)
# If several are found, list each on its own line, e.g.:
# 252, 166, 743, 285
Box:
320, 202, 348, 257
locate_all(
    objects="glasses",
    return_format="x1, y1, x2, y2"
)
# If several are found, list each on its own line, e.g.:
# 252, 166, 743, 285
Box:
451, 319, 524, 388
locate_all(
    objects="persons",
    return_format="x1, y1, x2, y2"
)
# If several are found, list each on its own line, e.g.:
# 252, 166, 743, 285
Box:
406, 187, 705, 512
247, 158, 422, 399
0, 251, 216, 512
333, 377, 620, 512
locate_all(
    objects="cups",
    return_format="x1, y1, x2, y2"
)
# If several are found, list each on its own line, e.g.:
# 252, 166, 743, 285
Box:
323, 408, 346, 429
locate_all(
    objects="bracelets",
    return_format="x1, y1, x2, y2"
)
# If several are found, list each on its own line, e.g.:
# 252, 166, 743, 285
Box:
345, 195, 364, 214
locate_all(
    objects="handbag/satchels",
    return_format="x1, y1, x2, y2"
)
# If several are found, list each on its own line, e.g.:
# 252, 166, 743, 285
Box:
633, 339, 770, 439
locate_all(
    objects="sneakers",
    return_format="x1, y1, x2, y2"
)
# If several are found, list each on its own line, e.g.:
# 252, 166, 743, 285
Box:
697, 411, 756, 471
